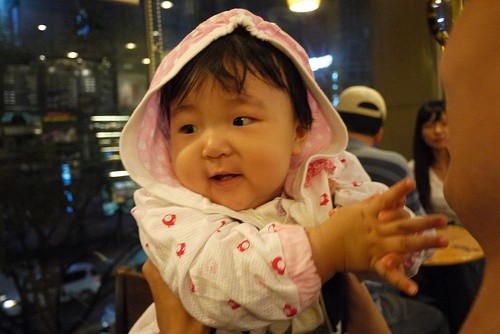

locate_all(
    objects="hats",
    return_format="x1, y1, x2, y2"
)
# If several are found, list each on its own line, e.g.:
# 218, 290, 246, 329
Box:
336, 85, 387, 119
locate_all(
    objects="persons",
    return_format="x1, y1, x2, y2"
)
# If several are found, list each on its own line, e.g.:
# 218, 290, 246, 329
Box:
336, 84, 446, 333
142, 0, 500, 333
118, 6, 449, 334
405, 99, 485, 301
